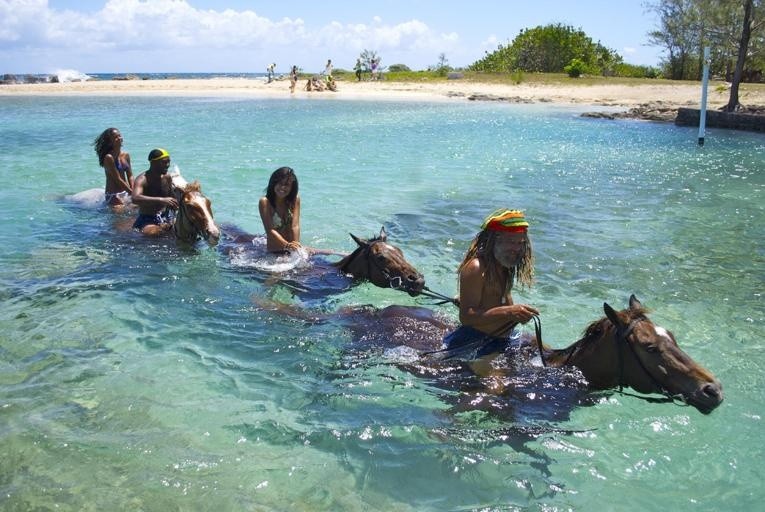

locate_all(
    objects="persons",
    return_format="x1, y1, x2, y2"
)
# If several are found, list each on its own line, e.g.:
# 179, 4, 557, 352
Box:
131, 147, 180, 238
370, 58, 378, 81
428, 209, 541, 445
251, 165, 320, 326
94, 127, 136, 214
288, 59, 337, 93
352, 59, 362, 81
267, 63, 276, 82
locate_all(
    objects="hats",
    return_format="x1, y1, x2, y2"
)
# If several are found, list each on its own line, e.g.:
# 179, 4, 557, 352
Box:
481, 209, 529, 234
148, 149, 169, 162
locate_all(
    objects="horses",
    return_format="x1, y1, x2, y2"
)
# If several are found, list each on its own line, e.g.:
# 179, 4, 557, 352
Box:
205, 222, 425, 297
36, 163, 189, 210
109, 179, 221, 256
242, 295, 725, 429
